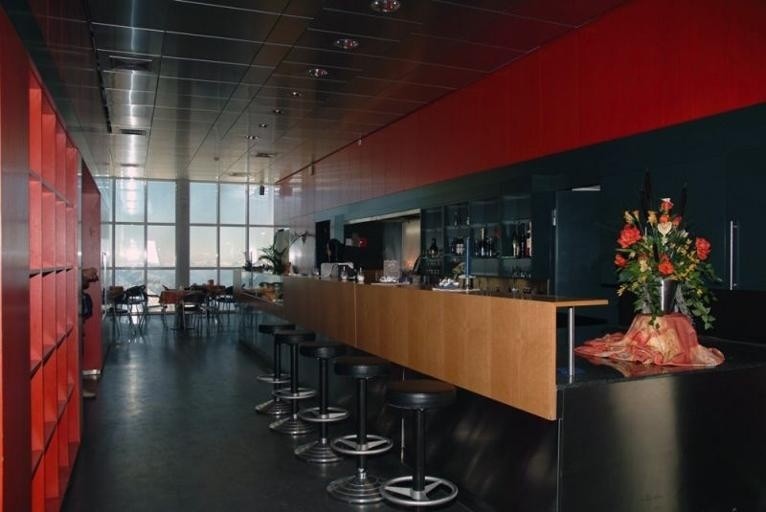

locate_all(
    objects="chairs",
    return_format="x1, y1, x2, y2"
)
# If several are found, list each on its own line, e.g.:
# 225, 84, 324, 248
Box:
98, 275, 241, 343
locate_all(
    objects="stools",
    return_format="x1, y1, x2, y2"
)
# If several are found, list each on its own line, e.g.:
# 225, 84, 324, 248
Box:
291, 340, 347, 462
268, 326, 317, 436
329, 356, 396, 506
253, 315, 293, 418
373, 379, 465, 510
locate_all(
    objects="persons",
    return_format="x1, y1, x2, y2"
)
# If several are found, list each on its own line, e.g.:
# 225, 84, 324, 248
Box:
82, 268, 99, 400
326, 238, 341, 263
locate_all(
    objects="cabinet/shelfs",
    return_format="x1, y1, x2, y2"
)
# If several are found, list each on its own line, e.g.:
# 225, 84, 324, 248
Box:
1, 4, 82, 511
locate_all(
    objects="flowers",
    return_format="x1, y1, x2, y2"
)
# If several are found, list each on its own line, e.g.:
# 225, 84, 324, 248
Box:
606, 195, 725, 333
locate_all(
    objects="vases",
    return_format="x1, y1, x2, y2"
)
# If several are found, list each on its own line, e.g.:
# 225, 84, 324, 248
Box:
647, 280, 680, 313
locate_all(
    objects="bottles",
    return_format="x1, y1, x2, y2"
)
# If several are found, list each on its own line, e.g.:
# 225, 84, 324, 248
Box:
471, 226, 495, 256
421, 237, 441, 288
510, 220, 532, 257
339, 266, 365, 282
448, 205, 467, 256
